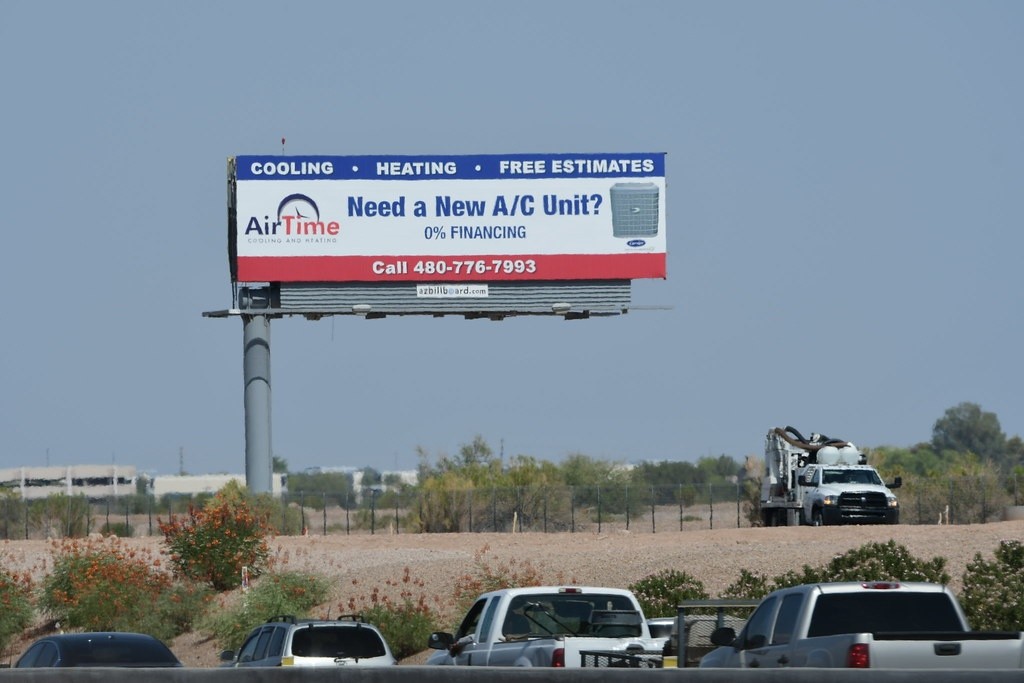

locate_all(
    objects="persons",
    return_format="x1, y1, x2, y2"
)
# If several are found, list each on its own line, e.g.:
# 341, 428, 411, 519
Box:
447, 633, 478, 657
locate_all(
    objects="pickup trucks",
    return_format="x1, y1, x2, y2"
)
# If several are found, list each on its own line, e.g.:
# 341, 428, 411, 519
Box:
700, 581, 1024, 668
423, 586, 669, 667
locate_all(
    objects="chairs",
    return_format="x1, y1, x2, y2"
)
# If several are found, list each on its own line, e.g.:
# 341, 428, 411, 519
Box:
502, 612, 531, 638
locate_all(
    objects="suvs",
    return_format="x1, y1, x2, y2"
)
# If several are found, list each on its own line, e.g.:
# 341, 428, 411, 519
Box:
218, 614, 400, 667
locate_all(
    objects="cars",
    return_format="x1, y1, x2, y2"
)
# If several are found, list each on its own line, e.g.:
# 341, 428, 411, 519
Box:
13, 632, 184, 667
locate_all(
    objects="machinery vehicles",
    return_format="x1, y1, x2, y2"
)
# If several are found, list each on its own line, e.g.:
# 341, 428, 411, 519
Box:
760, 425, 902, 526
